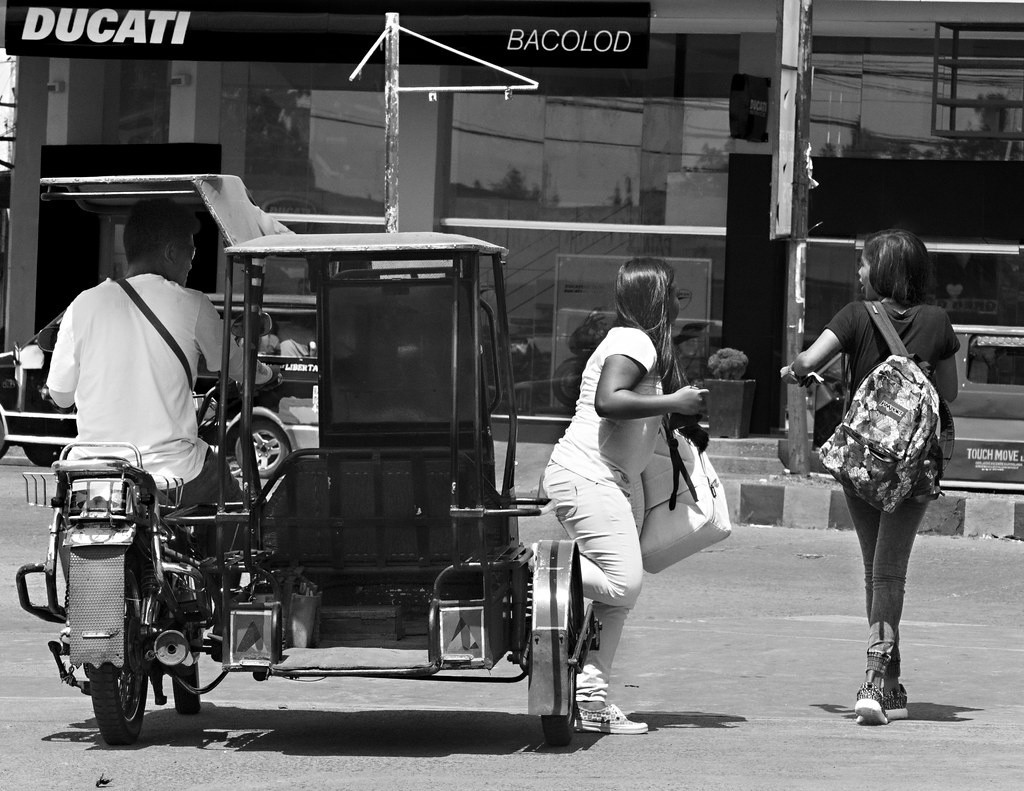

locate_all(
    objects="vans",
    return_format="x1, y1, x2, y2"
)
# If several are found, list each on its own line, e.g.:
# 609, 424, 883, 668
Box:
0, 292, 323, 469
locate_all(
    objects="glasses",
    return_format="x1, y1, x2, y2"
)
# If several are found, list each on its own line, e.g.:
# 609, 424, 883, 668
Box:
176, 238, 196, 260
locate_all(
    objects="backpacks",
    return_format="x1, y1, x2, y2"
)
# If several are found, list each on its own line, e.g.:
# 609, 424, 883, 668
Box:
621, 408, 732, 574
817, 300, 955, 514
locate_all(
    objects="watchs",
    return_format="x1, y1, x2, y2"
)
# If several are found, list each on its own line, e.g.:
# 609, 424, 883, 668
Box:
788, 361, 807, 382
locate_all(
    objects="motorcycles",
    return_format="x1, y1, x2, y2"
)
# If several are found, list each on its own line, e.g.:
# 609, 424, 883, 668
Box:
13, 171, 607, 749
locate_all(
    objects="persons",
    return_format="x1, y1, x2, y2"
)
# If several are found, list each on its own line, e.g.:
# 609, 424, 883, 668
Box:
527, 256, 710, 736
780, 228, 960, 725
46, 196, 280, 662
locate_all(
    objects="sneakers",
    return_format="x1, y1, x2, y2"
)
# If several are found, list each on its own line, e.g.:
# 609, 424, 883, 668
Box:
855, 682, 888, 725
882, 684, 909, 719
574, 704, 648, 734
